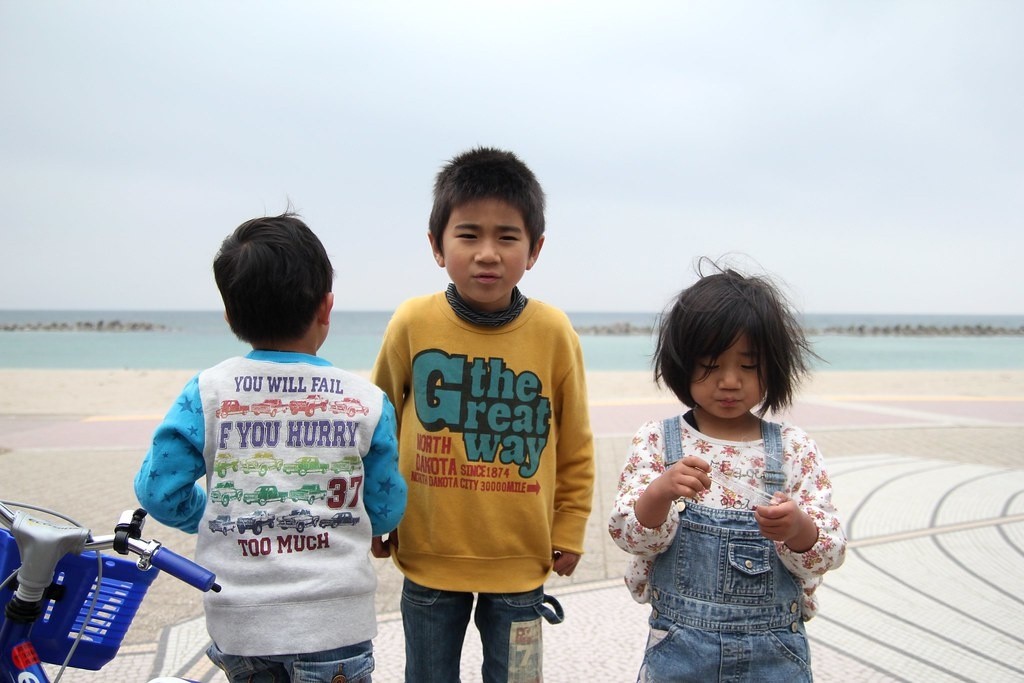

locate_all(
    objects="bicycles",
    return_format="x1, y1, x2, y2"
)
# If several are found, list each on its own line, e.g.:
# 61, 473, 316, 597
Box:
0, 495, 223, 683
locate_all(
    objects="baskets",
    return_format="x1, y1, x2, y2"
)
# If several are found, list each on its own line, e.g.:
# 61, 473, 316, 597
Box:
0, 526, 160, 672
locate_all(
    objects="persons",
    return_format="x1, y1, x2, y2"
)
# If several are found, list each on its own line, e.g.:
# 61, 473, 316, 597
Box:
369, 149, 596, 683
607, 267, 847, 683
133, 213, 407, 683
